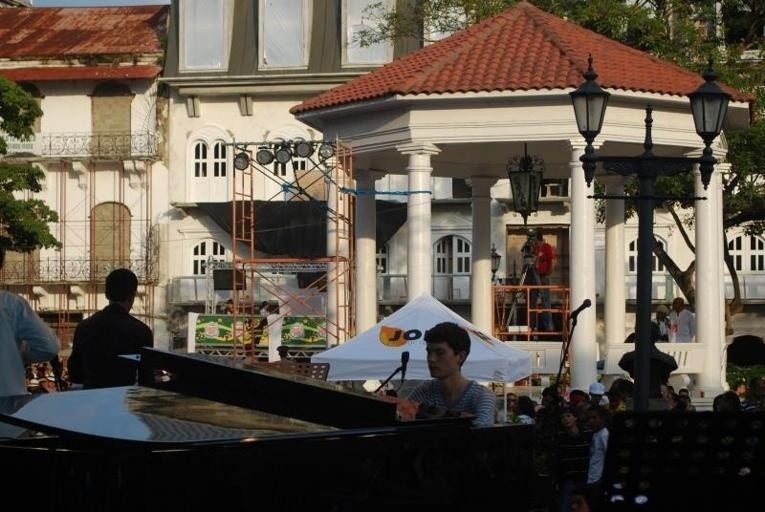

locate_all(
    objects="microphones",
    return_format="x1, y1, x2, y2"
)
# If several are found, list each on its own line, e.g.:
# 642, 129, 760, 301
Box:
568, 298, 592, 320
401, 351, 409, 384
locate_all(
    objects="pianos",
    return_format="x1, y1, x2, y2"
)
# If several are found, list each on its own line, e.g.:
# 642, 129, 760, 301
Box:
0, 346, 537, 511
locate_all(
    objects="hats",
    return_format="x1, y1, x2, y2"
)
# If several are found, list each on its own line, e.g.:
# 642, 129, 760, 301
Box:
589, 383, 604, 396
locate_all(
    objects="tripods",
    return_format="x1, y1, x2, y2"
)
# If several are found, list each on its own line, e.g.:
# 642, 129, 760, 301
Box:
504, 257, 555, 332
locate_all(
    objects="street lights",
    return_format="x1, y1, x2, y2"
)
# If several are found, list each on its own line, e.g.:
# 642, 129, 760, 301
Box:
488, 242, 502, 284
561, 46, 737, 507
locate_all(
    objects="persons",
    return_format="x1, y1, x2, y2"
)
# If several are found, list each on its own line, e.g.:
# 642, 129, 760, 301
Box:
505, 376, 764, 512
0, 242, 62, 440
651, 303, 674, 345
668, 298, 696, 343
523, 227, 555, 333
409, 321, 497, 427
67, 268, 154, 388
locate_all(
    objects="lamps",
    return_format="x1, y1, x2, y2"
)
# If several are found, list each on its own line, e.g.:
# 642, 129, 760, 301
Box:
233, 140, 334, 171
505, 142, 546, 227
